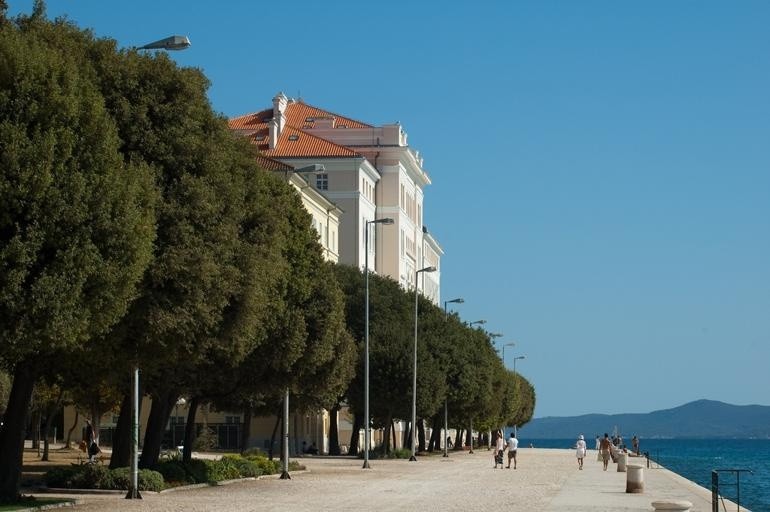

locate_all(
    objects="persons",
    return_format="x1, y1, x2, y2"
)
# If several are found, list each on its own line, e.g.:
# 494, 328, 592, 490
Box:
494, 432, 508, 469
86, 420, 95, 464
504, 432, 518, 469
576, 432, 639, 471
303, 440, 319, 455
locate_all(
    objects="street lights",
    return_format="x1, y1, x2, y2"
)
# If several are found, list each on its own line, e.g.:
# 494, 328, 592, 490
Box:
127, 31, 193, 499
278, 160, 329, 484
174, 396, 187, 422
406, 265, 527, 468
360, 215, 395, 470
